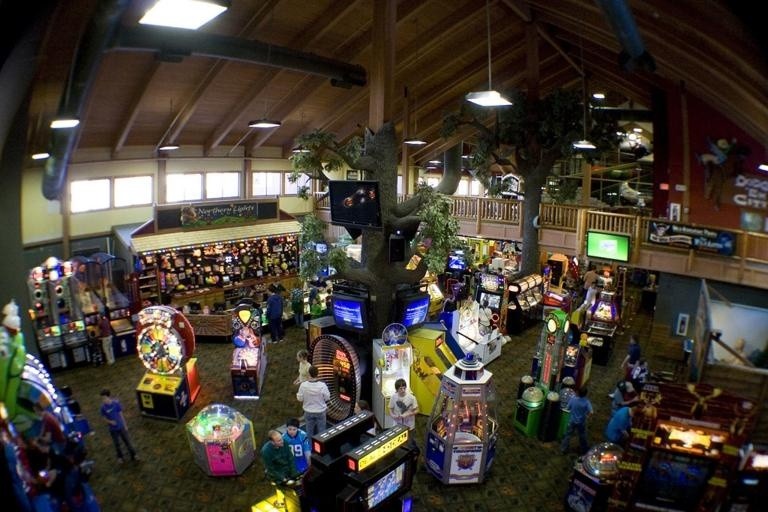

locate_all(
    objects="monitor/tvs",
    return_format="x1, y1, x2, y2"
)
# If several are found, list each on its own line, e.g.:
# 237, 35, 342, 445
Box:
447, 254, 467, 273
586, 228, 631, 263
327, 180, 383, 229
331, 293, 367, 332
396, 296, 431, 328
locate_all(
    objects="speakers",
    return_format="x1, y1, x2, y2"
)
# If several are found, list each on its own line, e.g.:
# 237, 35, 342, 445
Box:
387, 235, 408, 263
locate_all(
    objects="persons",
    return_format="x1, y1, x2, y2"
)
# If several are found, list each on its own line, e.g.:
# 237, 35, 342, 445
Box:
557, 383, 595, 456
577, 265, 600, 308
609, 379, 641, 409
719, 336, 747, 367
442, 291, 456, 313
32, 399, 63, 449
265, 281, 332, 344
599, 270, 612, 290
98, 387, 140, 464
478, 298, 493, 329
584, 281, 603, 304
386, 377, 420, 441
260, 429, 302, 489
463, 293, 476, 305
354, 398, 376, 437
473, 263, 486, 300
699, 129, 737, 213
456, 278, 466, 300
604, 404, 640, 445
297, 364, 331, 437
294, 349, 312, 387
25, 431, 49, 478
621, 333, 641, 380
281, 418, 313, 474
93, 311, 117, 366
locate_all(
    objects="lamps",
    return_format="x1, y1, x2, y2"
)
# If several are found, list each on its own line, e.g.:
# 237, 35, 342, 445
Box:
572, 69, 598, 149
158, 5, 427, 153
465, 6, 515, 109
